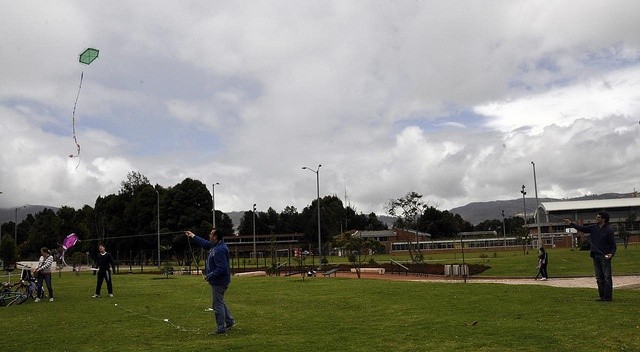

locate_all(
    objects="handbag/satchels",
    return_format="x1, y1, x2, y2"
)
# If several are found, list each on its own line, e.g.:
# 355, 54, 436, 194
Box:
33, 268, 44, 277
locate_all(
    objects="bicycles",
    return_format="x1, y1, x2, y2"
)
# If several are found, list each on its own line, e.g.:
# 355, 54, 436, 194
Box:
15, 277, 44, 301
0, 283, 27, 306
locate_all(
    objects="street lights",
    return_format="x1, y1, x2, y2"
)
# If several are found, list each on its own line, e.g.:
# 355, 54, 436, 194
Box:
501, 210, 506, 250
520, 185, 529, 254
252, 204, 256, 265
212, 183, 219, 229
14, 206, 27, 261
301, 165, 322, 270
154, 190, 160, 266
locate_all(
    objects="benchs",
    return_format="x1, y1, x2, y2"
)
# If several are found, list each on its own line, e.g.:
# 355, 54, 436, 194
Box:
322, 267, 339, 277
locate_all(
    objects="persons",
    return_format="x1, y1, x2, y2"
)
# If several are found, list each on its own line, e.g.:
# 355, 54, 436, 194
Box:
33, 247, 54, 303
562, 212, 617, 301
539, 247, 548, 280
183, 228, 236, 334
91, 243, 116, 298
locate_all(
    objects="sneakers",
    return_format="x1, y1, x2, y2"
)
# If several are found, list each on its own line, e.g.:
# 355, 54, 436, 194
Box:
50, 298, 54, 301
540, 277, 546, 281
92, 295, 100, 298
225, 322, 235, 330
211, 332, 223, 335
204, 308, 213, 312
35, 298, 40, 303
595, 299, 601, 301
601, 298, 612, 301
109, 294, 113, 297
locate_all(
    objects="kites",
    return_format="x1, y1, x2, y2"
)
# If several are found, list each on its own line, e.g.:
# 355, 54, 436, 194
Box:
79, 48, 99, 65
62, 233, 78, 250
